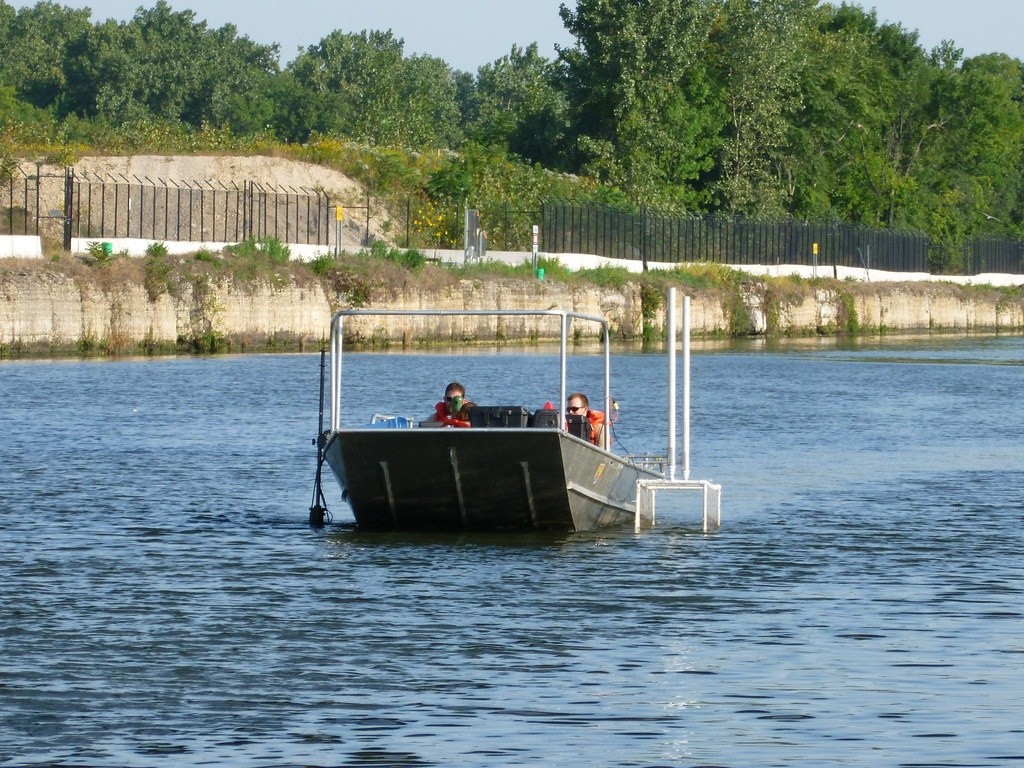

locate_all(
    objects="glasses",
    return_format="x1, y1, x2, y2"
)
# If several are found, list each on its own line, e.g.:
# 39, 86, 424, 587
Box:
443, 396, 452, 402
566, 406, 585, 413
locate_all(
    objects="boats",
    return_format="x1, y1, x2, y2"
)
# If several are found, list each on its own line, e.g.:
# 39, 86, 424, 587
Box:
309, 308, 666, 543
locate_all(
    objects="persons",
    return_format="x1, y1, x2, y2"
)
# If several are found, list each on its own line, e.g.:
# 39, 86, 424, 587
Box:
566, 392, 605, 450
426, 381, 478, 428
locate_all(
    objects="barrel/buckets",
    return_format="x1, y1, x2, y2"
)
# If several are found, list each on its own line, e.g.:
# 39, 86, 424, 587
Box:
565, 268, 570, 274
536, 269, 544, 279
101, 242, 112, 255
